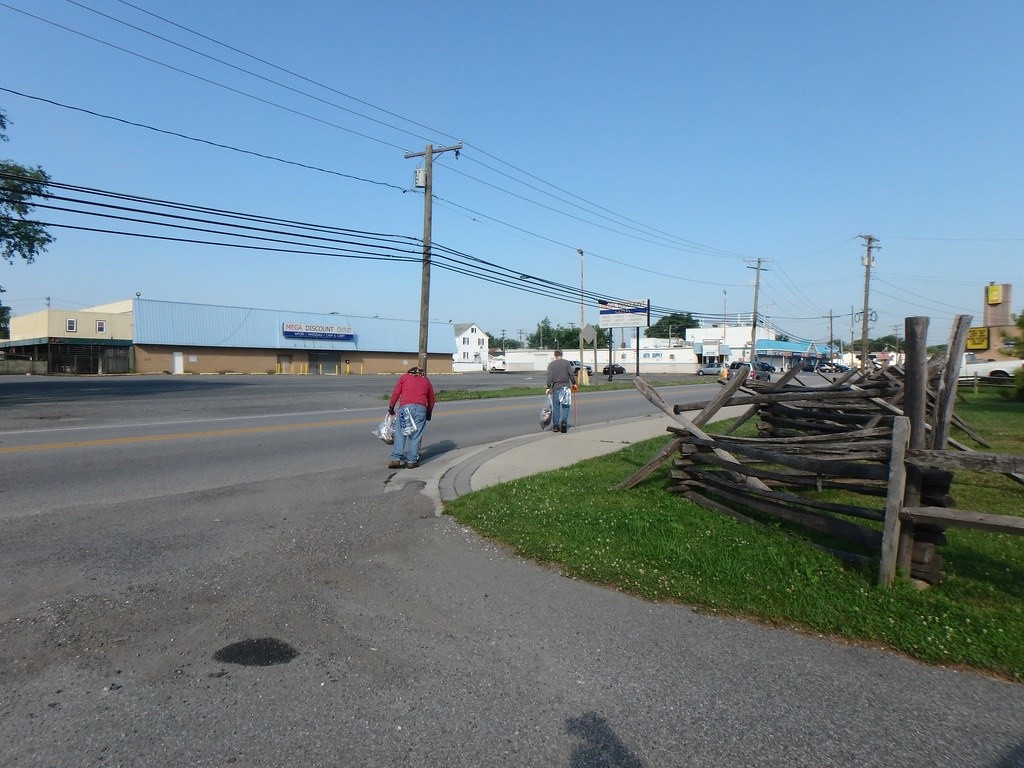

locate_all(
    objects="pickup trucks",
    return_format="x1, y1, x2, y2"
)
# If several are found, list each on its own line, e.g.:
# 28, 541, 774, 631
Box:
958, 352, 1024, 384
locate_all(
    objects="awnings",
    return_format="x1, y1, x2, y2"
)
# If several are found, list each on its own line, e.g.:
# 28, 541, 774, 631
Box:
693, 342, 732, 355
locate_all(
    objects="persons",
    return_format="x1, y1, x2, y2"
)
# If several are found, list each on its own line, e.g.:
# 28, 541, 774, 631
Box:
388, 367, 436, 469
545, 350, 578, 433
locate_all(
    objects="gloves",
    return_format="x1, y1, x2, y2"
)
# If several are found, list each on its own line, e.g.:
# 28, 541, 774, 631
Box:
388, 408, 396, 415
572, 384, 577, 392
426, 414, 431, 421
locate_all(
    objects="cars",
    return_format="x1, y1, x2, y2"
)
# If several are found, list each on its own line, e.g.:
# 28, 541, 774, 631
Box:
568, 360, 592, 376
816, 364, 836, 373
799, 364, 814, 372
729, 361, 771, 382
828, 363, 851, 373
602, 364, 626, 375
697, 362, 730, 376
855, 350, 905, 369
488, 357, 505, 371
761, 362, 776, 373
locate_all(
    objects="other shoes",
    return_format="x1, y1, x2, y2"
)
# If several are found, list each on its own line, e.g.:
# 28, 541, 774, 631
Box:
388, 461, 400, 468
553, 425, 560, 432
561, 425, 566, 432
407, 462, 419, 468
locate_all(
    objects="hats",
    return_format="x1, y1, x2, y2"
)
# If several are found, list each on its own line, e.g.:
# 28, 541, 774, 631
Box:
554, 349, 562, 356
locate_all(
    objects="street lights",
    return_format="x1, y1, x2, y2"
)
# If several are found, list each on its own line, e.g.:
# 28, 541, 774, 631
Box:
721, 289, 726, 365
577, 248, 584, 370
